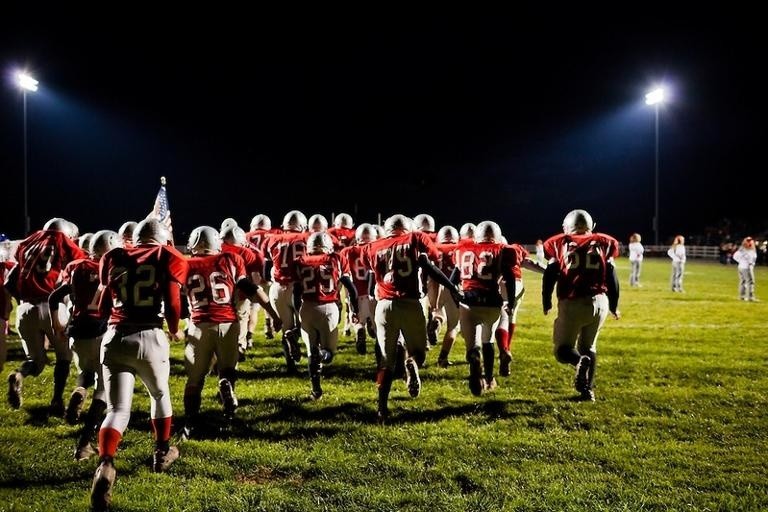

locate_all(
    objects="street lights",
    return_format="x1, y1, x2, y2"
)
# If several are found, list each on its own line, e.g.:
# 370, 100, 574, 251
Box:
640, 85, 673, 245
9, 63, 43, 242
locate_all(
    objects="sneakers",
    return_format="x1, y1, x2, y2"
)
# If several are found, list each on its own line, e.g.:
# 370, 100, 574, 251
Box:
91, 467, 115, 511
49, 396, 64, 419
74, 444, 100, 460
217, 320, 513, 421
155, 447, 179, 471
584, 392, 595, 400
64, 385, 86, 424
7, 373, 24, 408
180, 423, 197, 440
574, 354, 589, 389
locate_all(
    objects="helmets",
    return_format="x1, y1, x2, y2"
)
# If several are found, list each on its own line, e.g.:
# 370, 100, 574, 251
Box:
385, 214, 412, 238
309, 212, 354, 232
219, 217, 247, 245
414, 214, 435, 233
437, 221, 509, 246
305, 233, 335, 255
250, 214, 271, 231
281, 210, 307, 233
562, 208, 595, 235
355, 222, 383, 244
186, 225, 221, 253
42, 217, 174, 261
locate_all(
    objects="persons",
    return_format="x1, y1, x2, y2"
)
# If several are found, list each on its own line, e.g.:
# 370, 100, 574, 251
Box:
718, 234, 768, 266
86, 220, 180, 506
628, 233, 645, 288
1, 208, 547, 462
543, 210, 621, 402
732, 237, 761, 302
667, 235, 687, 293
